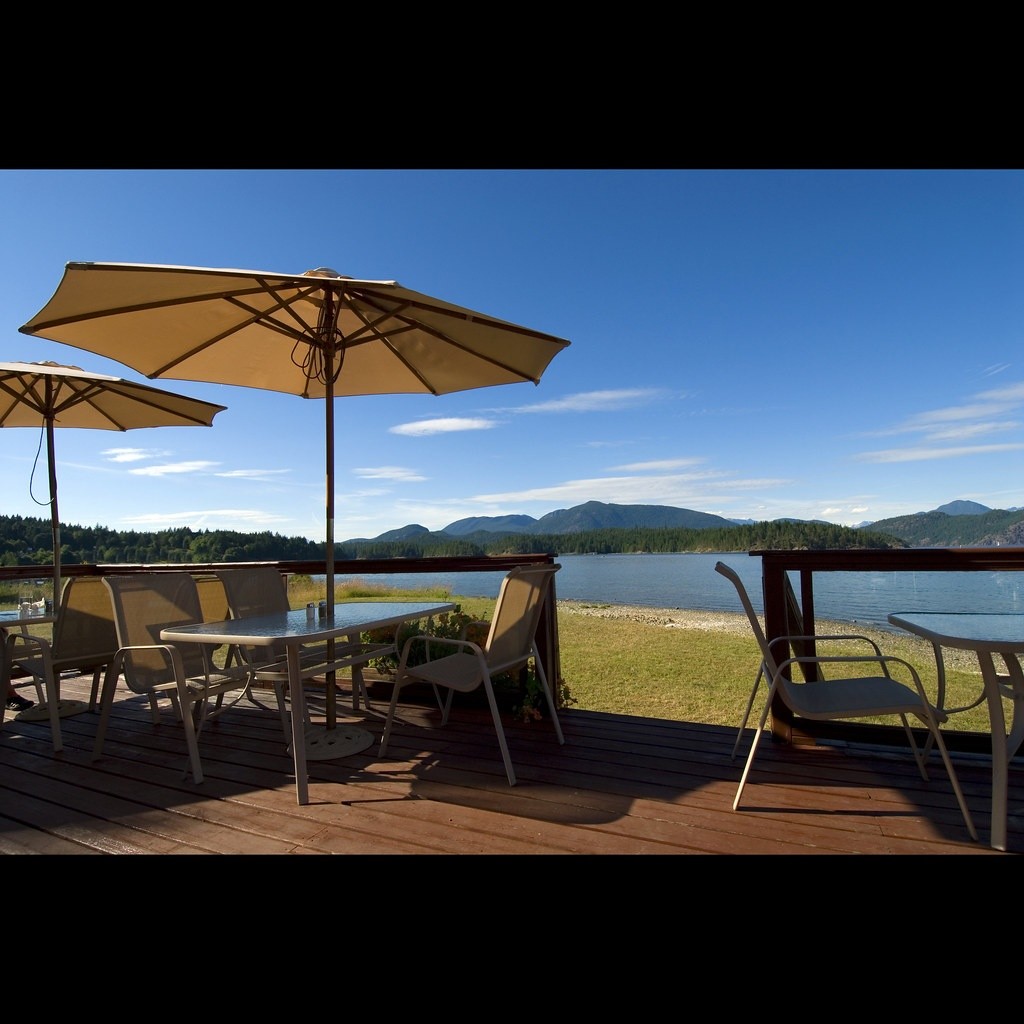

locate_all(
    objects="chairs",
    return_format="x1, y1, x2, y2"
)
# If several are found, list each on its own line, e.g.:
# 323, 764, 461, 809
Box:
0, 564, 566, 789
714, 562, 979, 841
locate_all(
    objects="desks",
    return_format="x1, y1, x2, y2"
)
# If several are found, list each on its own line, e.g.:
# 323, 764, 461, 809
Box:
887, 612, 1024, 852
0, 607, 59, 721
160, 600, 459, 807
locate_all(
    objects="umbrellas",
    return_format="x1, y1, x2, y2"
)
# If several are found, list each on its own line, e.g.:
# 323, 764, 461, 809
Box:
0, 362, 228, 704
18, 262, 572, 730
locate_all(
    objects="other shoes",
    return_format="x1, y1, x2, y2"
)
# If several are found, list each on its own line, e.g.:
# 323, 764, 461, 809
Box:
4, 696, 34, 711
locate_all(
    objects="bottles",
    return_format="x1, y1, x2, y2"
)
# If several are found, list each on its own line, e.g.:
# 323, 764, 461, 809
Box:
45, 599, 52, 612
318, 601, 327, 619
306, 602, 315, 620
